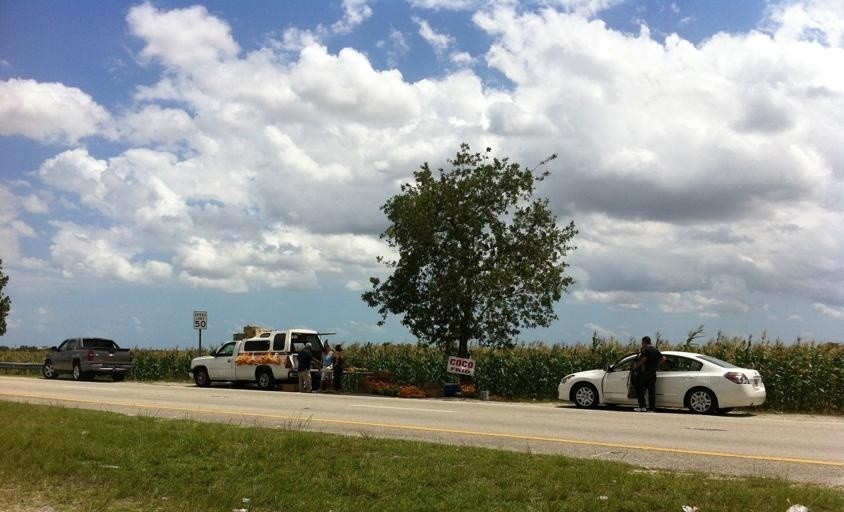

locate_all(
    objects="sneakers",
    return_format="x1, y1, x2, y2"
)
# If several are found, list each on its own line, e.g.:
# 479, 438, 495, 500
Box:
634, 408, 655, 413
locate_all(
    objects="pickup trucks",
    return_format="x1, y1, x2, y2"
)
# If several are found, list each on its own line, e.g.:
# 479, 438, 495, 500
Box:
186, 327, 338, 391
41, 336, 135, 383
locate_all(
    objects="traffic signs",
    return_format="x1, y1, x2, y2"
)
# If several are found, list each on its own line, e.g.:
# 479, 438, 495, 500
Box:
192, 310, 207, 330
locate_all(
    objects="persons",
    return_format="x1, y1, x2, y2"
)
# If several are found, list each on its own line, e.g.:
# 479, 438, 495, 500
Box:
330, 345, 345, 394
296, 342, 322, 392
625, 335, 668, 413
316, 343, 334, 393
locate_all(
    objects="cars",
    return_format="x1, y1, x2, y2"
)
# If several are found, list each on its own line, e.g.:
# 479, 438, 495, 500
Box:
556, 349, 767, 415
